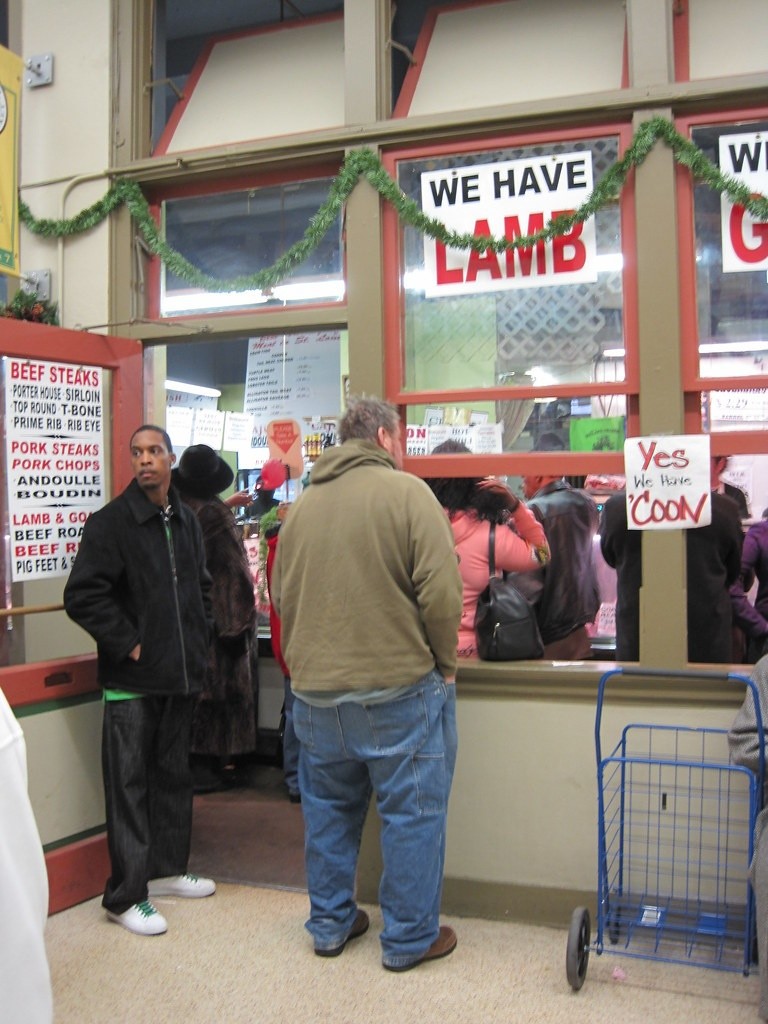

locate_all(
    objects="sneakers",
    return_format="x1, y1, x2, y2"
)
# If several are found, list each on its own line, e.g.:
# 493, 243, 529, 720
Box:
383, 925, 457, 972
146, 872, 216, 898
106, 899, 167, 935
314, 909, 369, 957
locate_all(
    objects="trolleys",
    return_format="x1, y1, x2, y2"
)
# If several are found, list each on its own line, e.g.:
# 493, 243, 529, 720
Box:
564, 666, 766, 991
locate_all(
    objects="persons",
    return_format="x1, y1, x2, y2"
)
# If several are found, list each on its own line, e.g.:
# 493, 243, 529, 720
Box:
421, 438, 551, 661
172, 443, 258, 791
600, 456, 747, 663
265, 398, 463, 974
502, 433, 600, 661
63, 425, 216, 935
728, 654, 768, 1024
730, 508, 768, 664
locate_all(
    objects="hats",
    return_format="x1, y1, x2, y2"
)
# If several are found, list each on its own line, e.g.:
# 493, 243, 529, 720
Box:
173, 443, 234, 498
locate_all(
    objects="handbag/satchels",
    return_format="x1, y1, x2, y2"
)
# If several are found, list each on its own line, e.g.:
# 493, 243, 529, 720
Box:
473, 517, 545, 662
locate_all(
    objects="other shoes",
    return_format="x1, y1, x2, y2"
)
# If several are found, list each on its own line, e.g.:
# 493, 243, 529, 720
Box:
289, 794, 302, 804
221, 767, 240, 785
